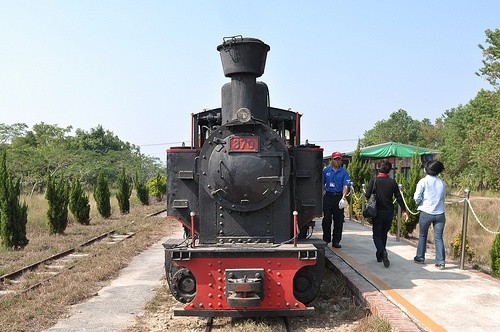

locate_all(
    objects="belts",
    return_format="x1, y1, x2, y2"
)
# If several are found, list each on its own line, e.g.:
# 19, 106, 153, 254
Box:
326, 192, 342, 196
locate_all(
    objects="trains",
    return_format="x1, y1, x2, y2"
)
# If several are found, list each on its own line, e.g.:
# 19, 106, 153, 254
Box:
161, 36, 328, 317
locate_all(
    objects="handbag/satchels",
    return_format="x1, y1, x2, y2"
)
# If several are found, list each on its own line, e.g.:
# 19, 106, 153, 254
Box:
363, 176, 377, 218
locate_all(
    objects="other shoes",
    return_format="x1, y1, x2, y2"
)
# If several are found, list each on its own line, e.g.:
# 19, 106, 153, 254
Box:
414, 257, 423, 264
435, 262, 445, 269
377, 257, 382, 262
382, 251, 389, 268
332, 243, 341, 248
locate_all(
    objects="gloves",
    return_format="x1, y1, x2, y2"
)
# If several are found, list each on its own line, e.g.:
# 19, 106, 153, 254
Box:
339, 197, 345, 209
321, 189, 326, 196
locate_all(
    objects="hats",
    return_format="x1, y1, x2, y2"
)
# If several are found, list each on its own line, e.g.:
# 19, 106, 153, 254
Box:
332, 152, 342, 160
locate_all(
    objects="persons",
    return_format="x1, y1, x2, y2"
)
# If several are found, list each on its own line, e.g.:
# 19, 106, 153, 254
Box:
364, 160, 409, 268
322, 152, 351, 248
414, 160, 447, 269
